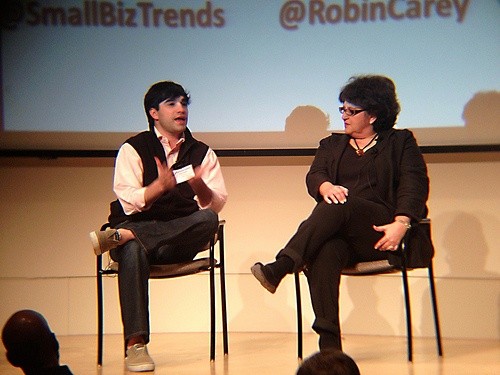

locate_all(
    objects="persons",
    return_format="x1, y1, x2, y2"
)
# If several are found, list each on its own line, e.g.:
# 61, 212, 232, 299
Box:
1, 309, 77, 375
250, 74, 430, 353
88, 79, 228, 372
296, 351, 360, 375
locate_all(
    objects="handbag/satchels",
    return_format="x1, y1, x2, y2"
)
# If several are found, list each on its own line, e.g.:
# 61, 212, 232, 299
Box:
386, 210, 435, 269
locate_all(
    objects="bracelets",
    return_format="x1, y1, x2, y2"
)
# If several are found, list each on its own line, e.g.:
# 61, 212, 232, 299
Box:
395, 219, 411, 229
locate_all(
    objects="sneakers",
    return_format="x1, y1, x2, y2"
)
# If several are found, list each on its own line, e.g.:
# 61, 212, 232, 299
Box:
251, 262, 284, 293
126, 343, 155, 371
89, 228, 122, 255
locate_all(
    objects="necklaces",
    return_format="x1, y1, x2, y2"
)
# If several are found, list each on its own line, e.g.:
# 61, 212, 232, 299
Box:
353, 134, 378, 156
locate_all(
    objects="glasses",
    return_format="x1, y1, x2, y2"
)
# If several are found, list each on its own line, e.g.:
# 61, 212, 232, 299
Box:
338, 106, 367, 116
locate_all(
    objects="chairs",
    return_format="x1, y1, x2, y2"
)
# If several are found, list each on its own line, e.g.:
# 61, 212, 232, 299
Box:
96, 220, 229, 369
293, 220, 444, 368
